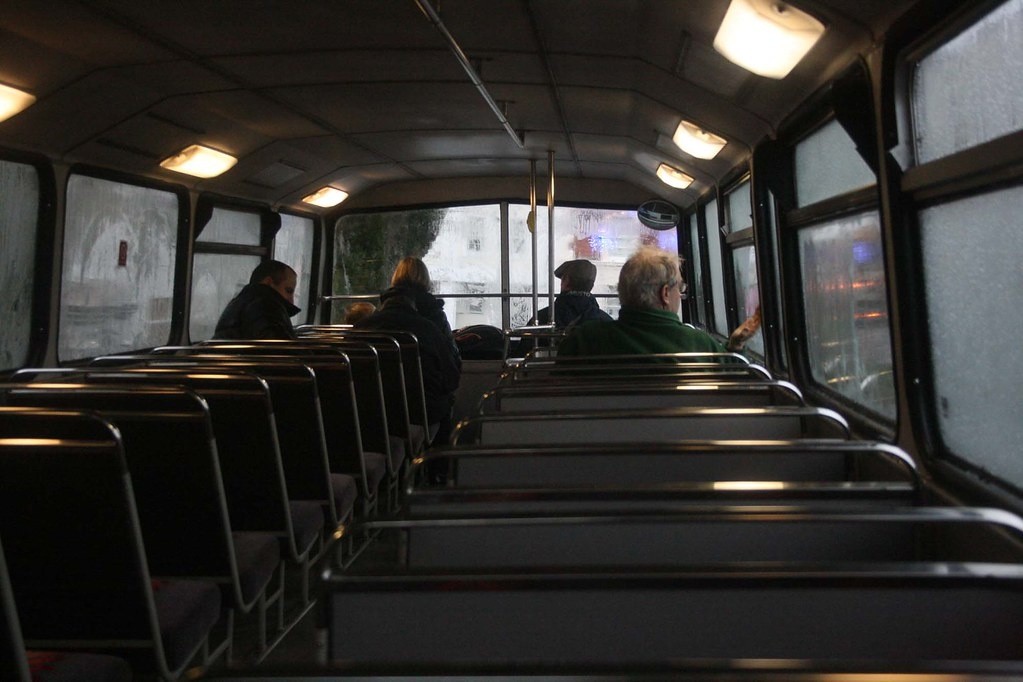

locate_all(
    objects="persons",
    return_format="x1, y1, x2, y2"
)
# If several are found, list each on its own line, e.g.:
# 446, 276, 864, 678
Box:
211, 258, 302, 340
346, 256, 462, 484
554, 257, 763, 376
528, 260, 613, 326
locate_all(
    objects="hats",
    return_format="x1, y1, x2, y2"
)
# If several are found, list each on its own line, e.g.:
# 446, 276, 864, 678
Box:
553, 259, 597, 281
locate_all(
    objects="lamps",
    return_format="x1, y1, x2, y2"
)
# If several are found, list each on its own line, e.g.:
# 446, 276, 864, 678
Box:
712, 0, 831, 81
301, 186, 349, 209
158, 143, 238, 179
656, 161, 695, 190
672, 120, 727, 160
0, 81, 38, 123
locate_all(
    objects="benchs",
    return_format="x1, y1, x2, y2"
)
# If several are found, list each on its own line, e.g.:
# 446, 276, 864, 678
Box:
0, 321, 451, 682
312, 346, 1023, 682
